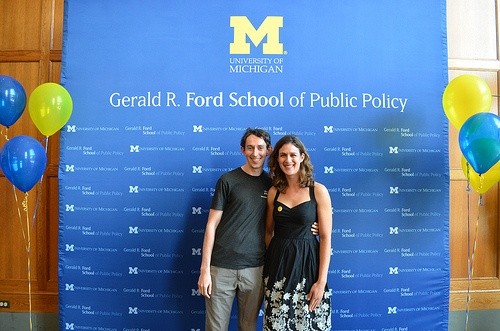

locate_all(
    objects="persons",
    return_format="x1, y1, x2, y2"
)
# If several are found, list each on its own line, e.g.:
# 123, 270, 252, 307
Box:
198, 127, 319, 331
263, 135, 332, 331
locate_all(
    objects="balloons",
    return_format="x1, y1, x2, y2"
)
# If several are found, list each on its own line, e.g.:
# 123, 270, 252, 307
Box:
0, 75, 74, 193
442, 74, 500, 195
